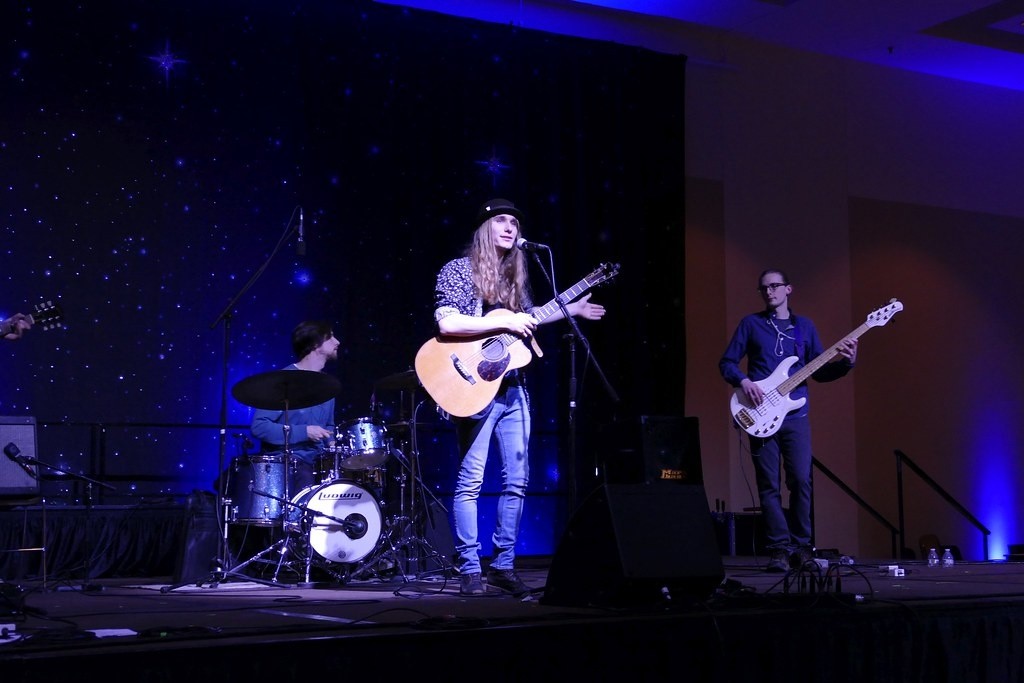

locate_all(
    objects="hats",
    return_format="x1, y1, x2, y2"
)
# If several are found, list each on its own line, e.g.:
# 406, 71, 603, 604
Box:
472, 199, 522, 227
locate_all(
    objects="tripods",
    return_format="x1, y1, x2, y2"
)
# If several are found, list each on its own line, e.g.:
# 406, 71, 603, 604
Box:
159, 226, 462, 594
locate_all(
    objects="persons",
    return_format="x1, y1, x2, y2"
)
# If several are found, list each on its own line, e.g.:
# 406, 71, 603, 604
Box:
0, 311, 34, 341
434, 199, 606, 596
251, 317, 347, 581
719, 269, 859, 571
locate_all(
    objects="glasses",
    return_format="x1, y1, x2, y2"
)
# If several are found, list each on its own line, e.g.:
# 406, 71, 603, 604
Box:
756, 283, 787, 293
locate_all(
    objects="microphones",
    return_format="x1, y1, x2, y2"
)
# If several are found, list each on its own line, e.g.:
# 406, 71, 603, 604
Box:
4, 442, 36, 478
296, 207, 305, 255
242, 436, 254, 449
516, 238, 549, 250
352, 519, 367, 534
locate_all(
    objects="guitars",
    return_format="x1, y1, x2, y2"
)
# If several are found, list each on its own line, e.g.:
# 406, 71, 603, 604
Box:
411, 261, 621, 425
0, 299, 66, 338
729, 297, 904, 439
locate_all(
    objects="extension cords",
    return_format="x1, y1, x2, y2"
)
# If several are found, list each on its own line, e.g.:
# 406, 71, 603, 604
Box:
0, 624, 16, 637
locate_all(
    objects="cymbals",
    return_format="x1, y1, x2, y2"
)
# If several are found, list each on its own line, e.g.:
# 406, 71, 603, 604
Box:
231, 369, 345, 412
385, 417, 430, 428
375, 369, 423, 392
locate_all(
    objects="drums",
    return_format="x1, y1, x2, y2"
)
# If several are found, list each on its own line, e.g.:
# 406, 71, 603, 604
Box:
280, 477, 383, 564
222, 453, 299, 528
312, 447, 359, 485
335, 416, 390, 471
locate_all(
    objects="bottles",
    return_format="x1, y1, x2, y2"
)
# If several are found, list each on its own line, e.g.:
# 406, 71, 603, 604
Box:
838, 556, 854, 565
941, 549, 954, 568
927, 548, 939, 568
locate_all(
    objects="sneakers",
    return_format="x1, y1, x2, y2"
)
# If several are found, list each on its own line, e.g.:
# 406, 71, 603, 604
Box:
767, 551, 789, 570
790, 549, 822, 569
486, 570, 535, 597
461, 573, 487, 596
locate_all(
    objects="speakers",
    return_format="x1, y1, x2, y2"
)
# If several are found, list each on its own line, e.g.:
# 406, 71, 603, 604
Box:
0, 416, 40, 496
546, 416, 725, 611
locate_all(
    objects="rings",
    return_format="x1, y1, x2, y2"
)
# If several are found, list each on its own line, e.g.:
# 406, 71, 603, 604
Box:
847, 347, 849, 349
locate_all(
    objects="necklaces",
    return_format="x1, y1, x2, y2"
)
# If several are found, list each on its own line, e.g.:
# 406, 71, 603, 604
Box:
768, 316, 796, 357
